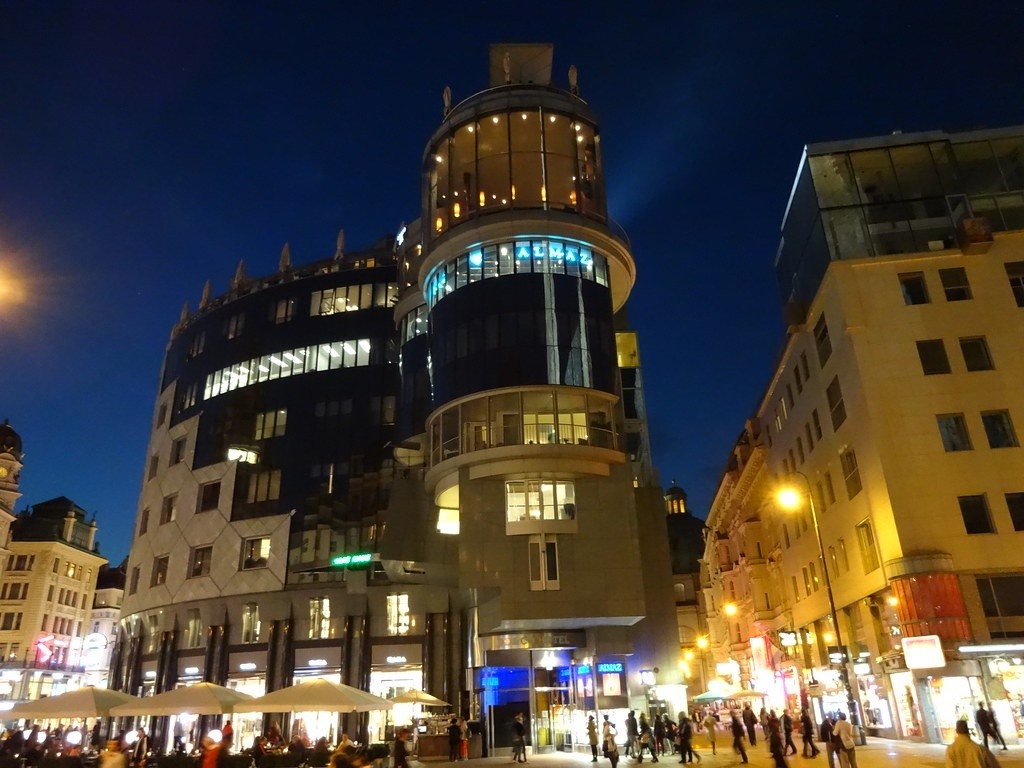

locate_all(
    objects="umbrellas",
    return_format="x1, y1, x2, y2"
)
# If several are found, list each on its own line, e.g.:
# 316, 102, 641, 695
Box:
388, 689, 451, 720
0, 685, 137, 719
234, 679, 393, 713
109, 682, 259, 716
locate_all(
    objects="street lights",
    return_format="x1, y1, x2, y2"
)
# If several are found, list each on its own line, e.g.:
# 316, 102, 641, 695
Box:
695, 636, 707, 692
778, 469, 869, 746
76, 632, 108, 689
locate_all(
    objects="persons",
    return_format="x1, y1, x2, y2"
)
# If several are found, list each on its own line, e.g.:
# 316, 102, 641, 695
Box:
873, 718, 877, 726
692, 707, 720, 755
910, 697, 921, 734
0, 720, 149, 768
945, 721, 1001, 768
797, 709, 820, 759
623, 710, 701, 765
586, 715, 620, 768
820, 711, 857, 768
960, 701, 1007, 750
760, 708, 797, 768
172, 718, 412, 768
448, 716, 470, 761
729, 703, 757, 763
1007, 693, 1024, 736
512, 709, 529, 763
